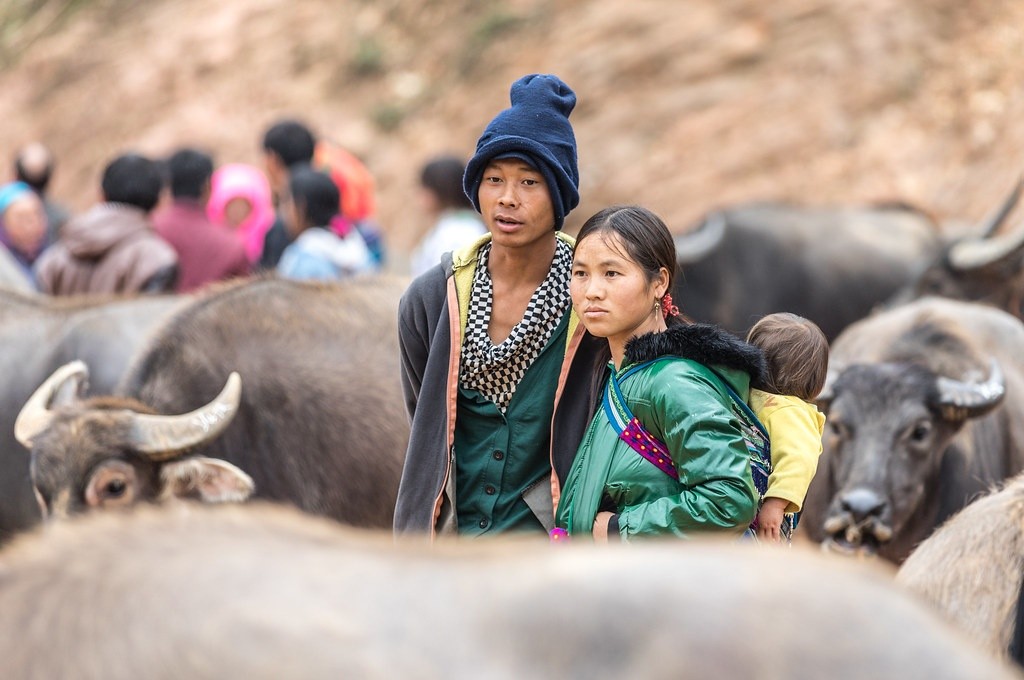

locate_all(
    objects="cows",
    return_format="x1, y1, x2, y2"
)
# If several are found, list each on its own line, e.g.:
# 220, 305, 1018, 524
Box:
0, 199, 1024, 680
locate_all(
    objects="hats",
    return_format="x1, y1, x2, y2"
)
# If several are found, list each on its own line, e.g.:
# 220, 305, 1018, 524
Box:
462, 73, 580, 231
0, 182, 30, 213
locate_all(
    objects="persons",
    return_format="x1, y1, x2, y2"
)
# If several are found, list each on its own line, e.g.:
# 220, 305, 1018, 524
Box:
0, 120, 490, 310
392, 74, 590, 541
555, 204, 833, 546
